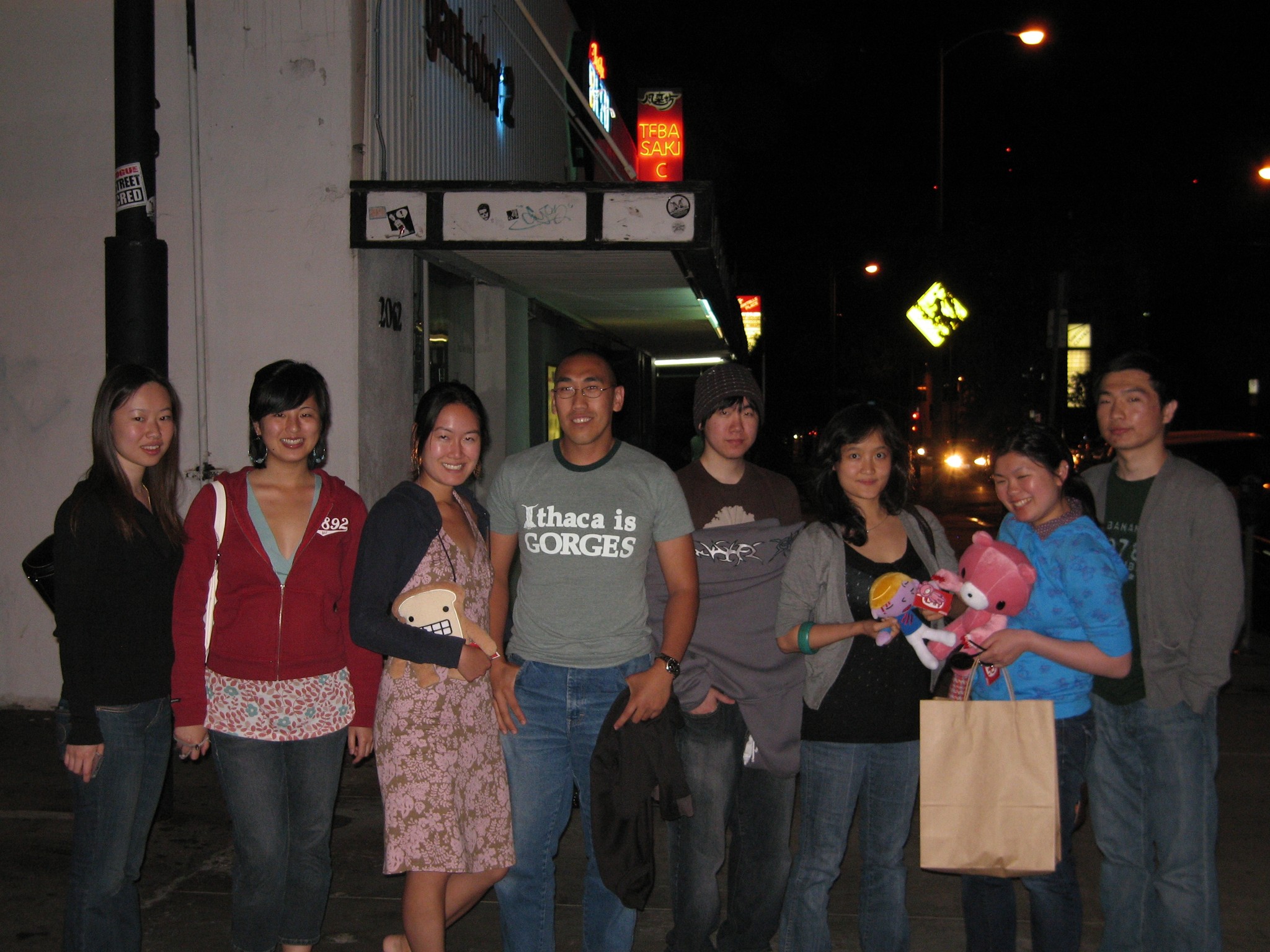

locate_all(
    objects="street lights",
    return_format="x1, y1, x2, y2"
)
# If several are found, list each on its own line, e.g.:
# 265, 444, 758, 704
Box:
830, 260, 880, 416
938, 20, 1051, 438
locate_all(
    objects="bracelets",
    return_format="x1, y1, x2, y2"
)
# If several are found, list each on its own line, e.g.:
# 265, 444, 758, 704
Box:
798, 622, 818, 656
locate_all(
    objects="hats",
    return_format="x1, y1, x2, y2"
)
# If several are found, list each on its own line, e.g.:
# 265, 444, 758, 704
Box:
694, 363, 764, 438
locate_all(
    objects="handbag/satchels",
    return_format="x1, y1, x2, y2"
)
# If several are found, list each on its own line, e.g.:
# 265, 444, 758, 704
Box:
919, 657, 1061, 878
22, 534, 57, 614
203, 480, 226, 664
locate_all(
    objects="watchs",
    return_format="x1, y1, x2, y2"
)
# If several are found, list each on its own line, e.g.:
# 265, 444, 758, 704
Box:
656, 652, 680, 679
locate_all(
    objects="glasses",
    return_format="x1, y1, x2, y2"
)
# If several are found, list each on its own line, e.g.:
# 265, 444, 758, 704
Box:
552, 385, 616, 399
947, 640, 993, 670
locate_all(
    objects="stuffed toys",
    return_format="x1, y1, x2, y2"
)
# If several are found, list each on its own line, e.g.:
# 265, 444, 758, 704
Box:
927, 528, 1034, 664
868, 574, 955, 669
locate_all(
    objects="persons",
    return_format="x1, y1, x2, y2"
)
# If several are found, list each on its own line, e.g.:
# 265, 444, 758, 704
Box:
50, 367, 189, 952
165, 361, 386, 952
950, 421, 1133, 952
632, 350, 813, 951
768, 391, 962, 952
1053, 355, 1247, 952
351, 375, 519, 951
476, 352, 698, 951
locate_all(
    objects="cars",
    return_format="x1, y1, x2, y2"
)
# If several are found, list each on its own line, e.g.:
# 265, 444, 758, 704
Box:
1100, 429, 1270, 626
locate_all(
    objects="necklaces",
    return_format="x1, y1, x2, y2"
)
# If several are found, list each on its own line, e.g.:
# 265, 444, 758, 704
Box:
864, 512, 892, 534
141, 482, 152, 512
